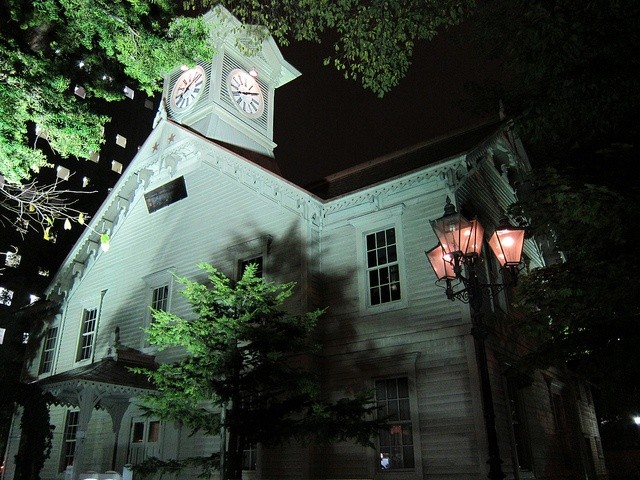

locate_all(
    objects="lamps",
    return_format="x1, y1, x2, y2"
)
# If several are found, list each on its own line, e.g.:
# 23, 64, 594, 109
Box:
428, 195, 473, 280
463, 215, 485, 264
481, 207, 526, 306
424, 241, 472, 305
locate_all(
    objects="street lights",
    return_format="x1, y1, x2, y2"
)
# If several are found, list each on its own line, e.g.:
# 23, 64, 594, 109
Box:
424, 194, 525, 475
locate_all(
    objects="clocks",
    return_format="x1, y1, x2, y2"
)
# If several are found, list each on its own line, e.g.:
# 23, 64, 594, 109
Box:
225, 68, 265, 119
170, 64, 206, 115
